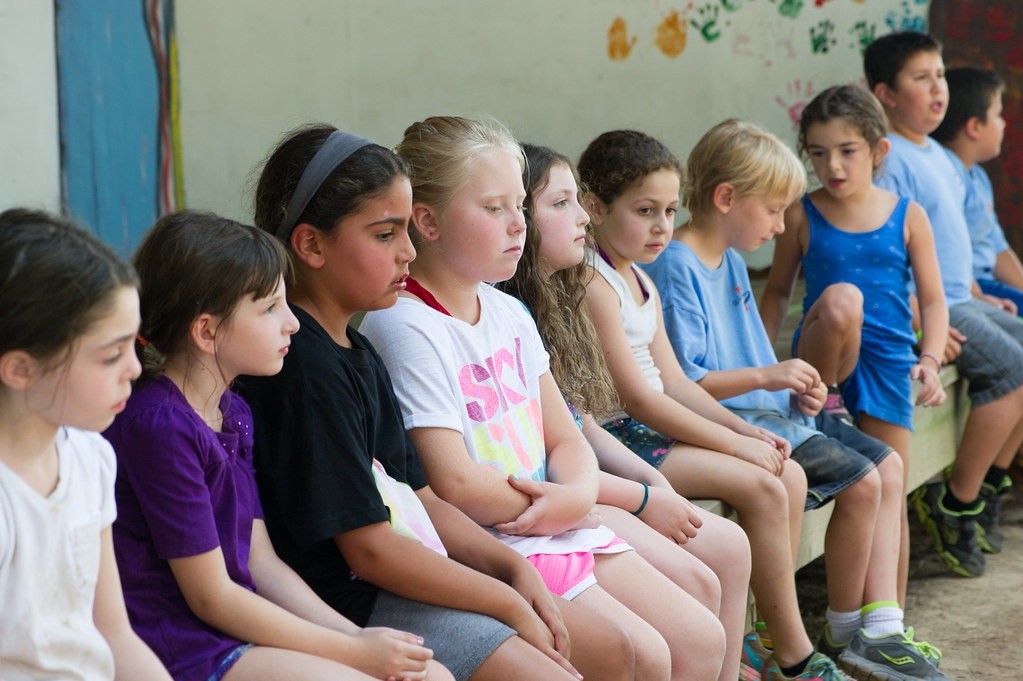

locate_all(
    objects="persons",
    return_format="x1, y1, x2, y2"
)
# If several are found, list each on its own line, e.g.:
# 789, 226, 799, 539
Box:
862, 29, 1021, 579
237, 123, 640, 680
376, 116, 730, 680
759, 81, 952, 626
566, 124, 848, 678
123, 206, 460, 681
490, 138, 754, 675
938, 61, 1021, 309
0, 206, 175, 680
636, 123, 955, 681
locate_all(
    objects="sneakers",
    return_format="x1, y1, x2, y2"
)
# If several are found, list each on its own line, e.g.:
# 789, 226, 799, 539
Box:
738, 630, 775, 681
823, 384, 856, 428
909, 481, 987, 577
761, 653, 858, 681
943, 460, 1013, 554
818, 623, 954, 681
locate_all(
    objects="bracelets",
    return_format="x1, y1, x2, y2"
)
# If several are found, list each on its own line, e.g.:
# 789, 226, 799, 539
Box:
632, 481, 649, 516
916, 352, 942, 372
